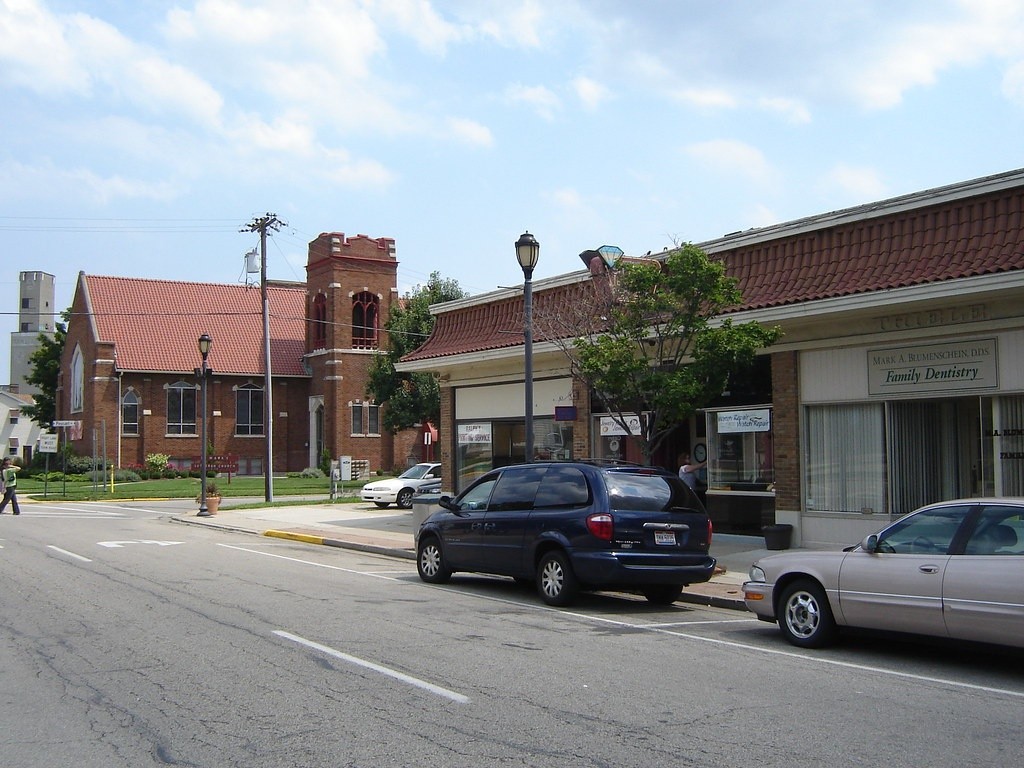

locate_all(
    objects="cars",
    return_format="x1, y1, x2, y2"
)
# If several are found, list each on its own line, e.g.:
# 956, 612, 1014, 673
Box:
359, 461, 442, 509
741, 494, 1024, 664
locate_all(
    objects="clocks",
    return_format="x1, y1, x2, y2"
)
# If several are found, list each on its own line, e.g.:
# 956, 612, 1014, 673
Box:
694, 443, 707, 464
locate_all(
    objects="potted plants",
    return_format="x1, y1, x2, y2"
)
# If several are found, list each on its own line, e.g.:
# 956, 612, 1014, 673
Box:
195, 480, 222, 516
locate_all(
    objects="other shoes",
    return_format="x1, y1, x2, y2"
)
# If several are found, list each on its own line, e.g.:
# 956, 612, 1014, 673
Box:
13, 511, 20, 515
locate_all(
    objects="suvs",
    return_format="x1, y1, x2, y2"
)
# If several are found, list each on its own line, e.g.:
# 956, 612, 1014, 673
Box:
413, 457, 717, 608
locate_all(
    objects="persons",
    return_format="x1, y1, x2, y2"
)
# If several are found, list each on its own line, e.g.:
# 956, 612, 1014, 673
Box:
677, 452, 705, 504
0, 456, 22, 515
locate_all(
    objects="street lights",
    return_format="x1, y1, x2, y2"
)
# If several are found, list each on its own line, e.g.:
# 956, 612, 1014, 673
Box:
514, 229, 540, 462
196, 332, 212, 516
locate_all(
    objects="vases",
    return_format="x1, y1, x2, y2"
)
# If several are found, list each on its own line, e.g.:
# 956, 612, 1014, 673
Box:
762, 524, 792, 550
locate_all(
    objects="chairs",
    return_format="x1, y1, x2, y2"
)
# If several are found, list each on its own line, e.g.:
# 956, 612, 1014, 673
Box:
982, 525, 1019, 556
